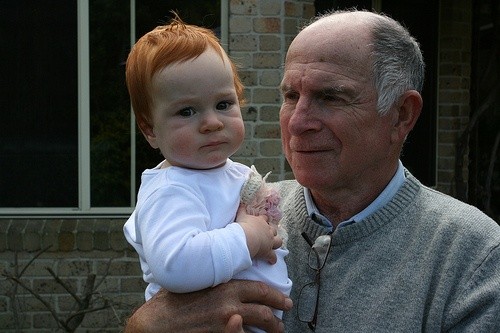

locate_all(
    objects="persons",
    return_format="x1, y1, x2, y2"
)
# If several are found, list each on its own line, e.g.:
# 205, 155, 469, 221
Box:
120, 8, 294, 332
121, 11, 499, 332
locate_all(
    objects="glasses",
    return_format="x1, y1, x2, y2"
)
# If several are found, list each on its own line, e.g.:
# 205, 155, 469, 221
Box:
296, 231, 334, 332
